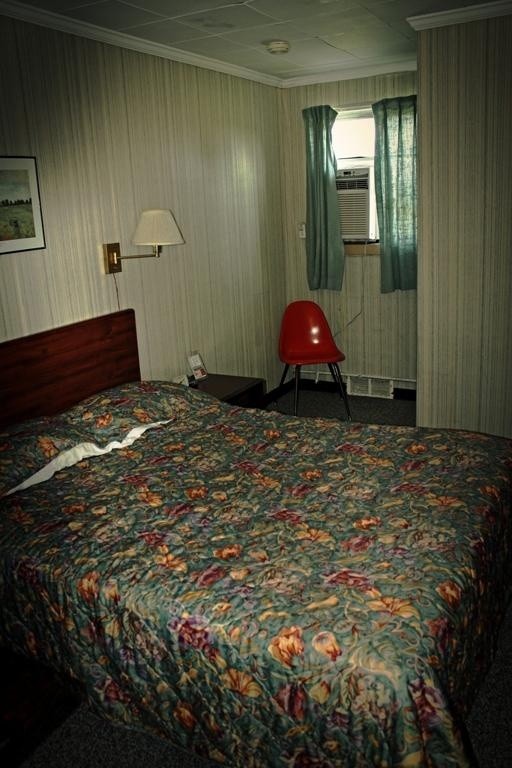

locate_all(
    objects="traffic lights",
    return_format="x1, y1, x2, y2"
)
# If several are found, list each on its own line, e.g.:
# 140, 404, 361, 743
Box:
0, 379, 221, 501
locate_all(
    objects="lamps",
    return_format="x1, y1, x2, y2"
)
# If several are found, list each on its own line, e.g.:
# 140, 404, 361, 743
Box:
278, 300, 353, 422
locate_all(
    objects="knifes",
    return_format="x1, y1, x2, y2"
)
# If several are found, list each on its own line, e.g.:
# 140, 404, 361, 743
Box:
336, 166, 380, 244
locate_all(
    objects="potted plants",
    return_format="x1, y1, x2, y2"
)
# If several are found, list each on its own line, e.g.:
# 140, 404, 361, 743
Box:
0, 155, 46, 255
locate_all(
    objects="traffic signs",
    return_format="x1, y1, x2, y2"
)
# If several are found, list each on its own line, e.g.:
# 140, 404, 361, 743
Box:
298, 224, 307, 239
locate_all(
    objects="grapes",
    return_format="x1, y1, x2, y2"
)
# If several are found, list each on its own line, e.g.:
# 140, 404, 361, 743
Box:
189, 373, 266, 411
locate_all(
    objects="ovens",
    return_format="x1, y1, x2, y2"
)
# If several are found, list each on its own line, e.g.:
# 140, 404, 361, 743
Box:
0, 308, 512, 768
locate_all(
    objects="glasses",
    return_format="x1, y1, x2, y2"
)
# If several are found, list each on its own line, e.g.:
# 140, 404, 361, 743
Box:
102, 208, 186, 275
